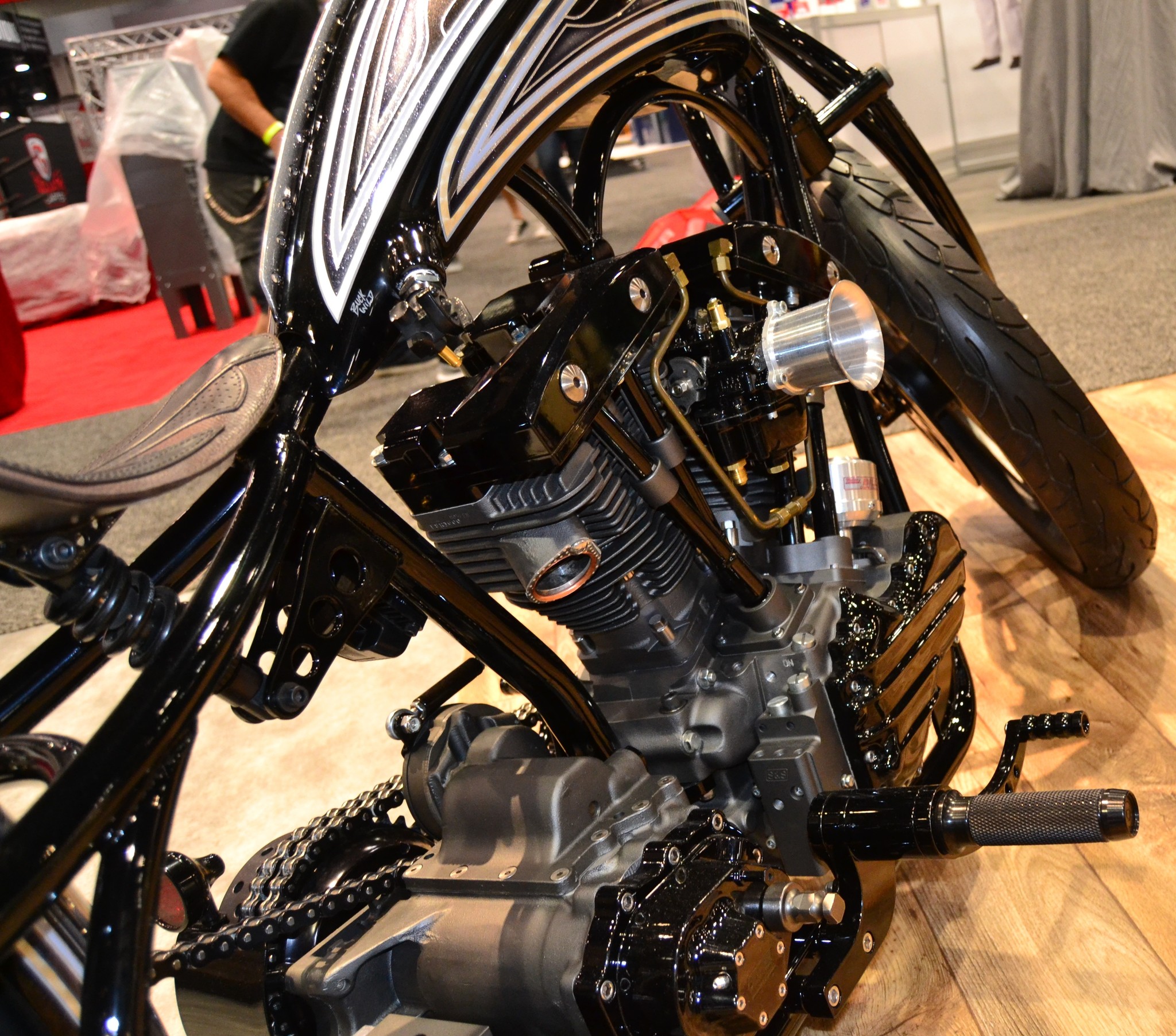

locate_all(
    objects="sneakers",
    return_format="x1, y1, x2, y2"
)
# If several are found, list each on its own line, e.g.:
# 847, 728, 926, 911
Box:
445, 254, 463, 273
533, 221, 552, 238
506, 217, 531, 243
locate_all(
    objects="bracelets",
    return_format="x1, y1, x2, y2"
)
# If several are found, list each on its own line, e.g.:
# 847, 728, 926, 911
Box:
262, 120, 286, 146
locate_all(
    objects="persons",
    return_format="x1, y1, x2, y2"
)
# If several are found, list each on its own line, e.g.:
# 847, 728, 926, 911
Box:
499, 186, 553, 244
967, 0, 1026, 69
536, 126, 589, 207
203, 1, 329, 348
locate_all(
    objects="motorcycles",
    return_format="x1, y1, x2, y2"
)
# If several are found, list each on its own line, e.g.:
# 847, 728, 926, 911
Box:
0, 0, 1166, 1034
